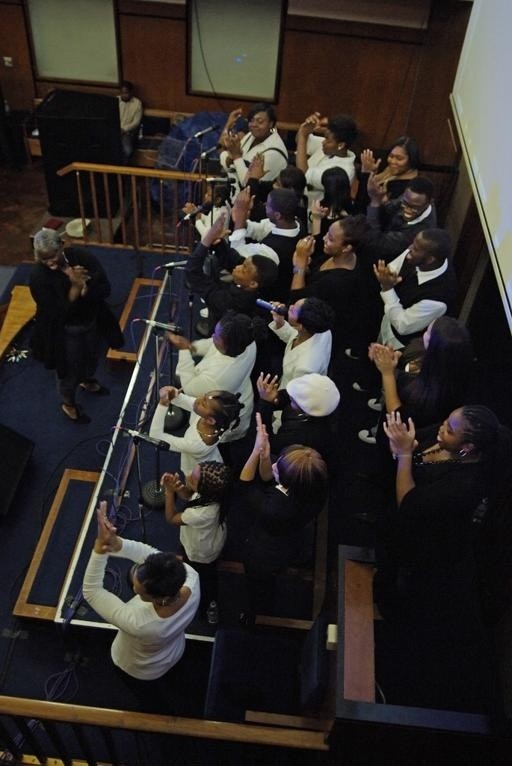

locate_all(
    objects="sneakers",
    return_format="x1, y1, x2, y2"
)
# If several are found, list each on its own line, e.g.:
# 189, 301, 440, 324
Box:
358, 429, 376, 444
368, 398, 382, 411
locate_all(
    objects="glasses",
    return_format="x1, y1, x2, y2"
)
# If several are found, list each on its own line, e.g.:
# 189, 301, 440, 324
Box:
399, 198, 427, 213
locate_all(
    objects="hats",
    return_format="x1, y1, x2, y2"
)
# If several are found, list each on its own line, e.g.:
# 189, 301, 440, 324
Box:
286, 373, 341, 417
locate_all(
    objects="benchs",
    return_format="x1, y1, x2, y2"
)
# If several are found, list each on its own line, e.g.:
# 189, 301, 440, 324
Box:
26, 98, 331, 198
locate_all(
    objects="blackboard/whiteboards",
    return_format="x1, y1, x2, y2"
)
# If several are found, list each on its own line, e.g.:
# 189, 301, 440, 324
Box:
186, 0, 288, 105
25, 0, 122, 88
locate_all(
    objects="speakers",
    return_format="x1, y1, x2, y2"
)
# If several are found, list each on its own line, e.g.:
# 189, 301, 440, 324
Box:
33, 88, 124, 219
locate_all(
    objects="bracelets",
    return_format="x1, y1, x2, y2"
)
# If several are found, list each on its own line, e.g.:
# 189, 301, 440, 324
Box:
394, 451, 417, 460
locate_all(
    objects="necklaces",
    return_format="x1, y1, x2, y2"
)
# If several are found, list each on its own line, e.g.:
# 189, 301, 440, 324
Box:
152, 593, 186, 608
197, 427, 220, 438
406, 448, 469, 466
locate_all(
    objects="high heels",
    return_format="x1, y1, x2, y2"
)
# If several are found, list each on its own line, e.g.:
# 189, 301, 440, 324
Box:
79, 381, 110, 396
60, 403, 91, 424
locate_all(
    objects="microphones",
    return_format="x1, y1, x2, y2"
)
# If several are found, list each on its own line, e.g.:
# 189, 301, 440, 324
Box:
193, 122, 221, 138
175, 201, 214, 229
133, 316, 186, 337
206, 177, 236, 184
120, 427, 170, 451
256, 298, 288, 315
160, 259, 188, 270
201, 143, 223, 160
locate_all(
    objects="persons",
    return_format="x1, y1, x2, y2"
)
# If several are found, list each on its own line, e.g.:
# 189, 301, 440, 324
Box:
236, 410, 330, 553
165, 103, 480, 444
146, 384, 244, 496
368, 401, 510, 580
80, 500, 202, 699
156, 459, 230, 626
27, 226, 127, 425
113, 79, 145, 135
